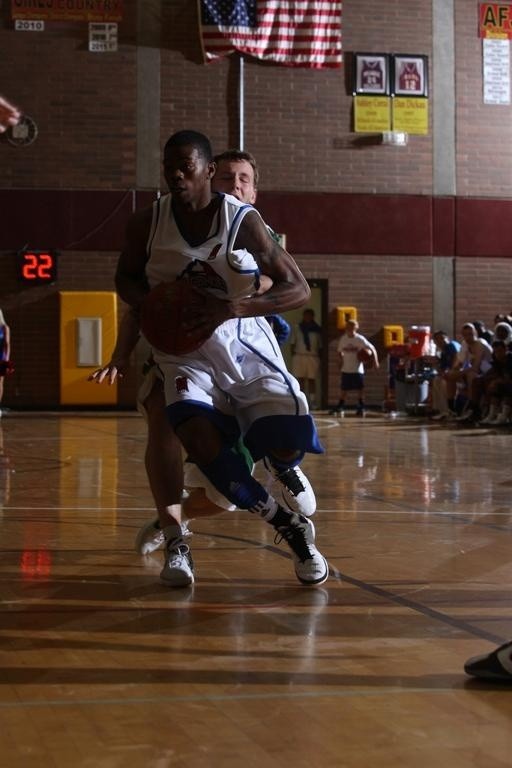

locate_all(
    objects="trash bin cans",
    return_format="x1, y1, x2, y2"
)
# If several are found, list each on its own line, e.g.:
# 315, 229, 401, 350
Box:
395, 375, 429, 411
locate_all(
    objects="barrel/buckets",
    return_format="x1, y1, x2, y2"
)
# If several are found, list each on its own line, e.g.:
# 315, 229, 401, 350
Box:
394, 380, 431, 412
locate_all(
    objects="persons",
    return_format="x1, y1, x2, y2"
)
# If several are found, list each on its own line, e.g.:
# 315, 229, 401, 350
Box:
0, 308, 10, 419
329, 320, 380, 416
429, 313, 512, 425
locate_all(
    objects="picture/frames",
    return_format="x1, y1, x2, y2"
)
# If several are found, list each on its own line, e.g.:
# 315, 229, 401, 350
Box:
350, 48, 430, 99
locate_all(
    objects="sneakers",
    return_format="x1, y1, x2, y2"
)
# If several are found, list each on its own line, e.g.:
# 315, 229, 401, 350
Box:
310, 401, 319, 411
431, 407, 512, 425
328, 407, 366, 418
264, 454, 329, 586
464, 639, 512, 679
135, 490, 195, 588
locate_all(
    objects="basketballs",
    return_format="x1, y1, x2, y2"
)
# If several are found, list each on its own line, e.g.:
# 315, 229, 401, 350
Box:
136, 280, 215, 356
357, 348, 373, 362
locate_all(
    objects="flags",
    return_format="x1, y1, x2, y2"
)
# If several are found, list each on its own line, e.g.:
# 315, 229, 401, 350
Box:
198, 1, 343, 70
